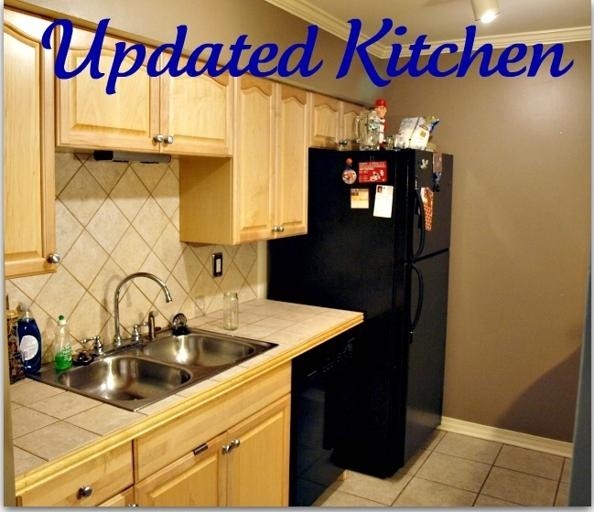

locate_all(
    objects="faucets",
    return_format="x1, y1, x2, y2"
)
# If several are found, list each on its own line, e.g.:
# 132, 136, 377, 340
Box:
112, 272, 173, 346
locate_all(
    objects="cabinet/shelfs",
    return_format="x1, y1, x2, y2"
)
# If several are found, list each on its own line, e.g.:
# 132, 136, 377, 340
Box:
4, 9, 54, 279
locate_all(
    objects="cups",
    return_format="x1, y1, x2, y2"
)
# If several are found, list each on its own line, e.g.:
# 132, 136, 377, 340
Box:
223, 292, 240, 332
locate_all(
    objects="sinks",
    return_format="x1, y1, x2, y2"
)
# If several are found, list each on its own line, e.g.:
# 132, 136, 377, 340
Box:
32, 320, 278, 413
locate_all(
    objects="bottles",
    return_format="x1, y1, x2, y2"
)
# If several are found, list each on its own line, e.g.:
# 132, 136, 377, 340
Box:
268, 148, 454, 476
52, 315, 74, 368
18, 302, 41, 374
355, 111, 379, 150
